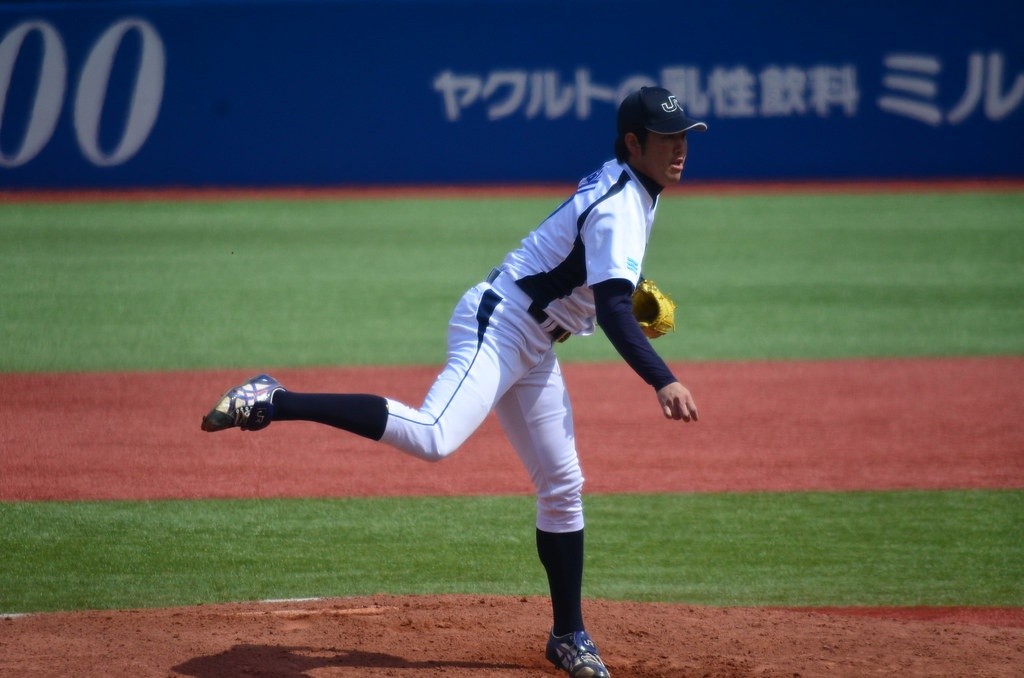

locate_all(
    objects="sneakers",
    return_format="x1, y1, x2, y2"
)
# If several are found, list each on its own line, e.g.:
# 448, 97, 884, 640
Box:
201, 373, 288, 432
546, 625, 610, 678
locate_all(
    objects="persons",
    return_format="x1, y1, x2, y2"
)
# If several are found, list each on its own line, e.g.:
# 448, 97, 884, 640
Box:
196, 85, 710, 678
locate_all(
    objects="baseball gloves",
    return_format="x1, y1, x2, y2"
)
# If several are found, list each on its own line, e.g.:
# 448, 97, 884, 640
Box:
632, 281, 675, 337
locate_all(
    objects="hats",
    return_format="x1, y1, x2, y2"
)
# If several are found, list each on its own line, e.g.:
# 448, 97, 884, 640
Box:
619, 86, 712, 135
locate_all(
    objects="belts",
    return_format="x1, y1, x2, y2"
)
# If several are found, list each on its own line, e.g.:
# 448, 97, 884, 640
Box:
485, 268, 571, 343
